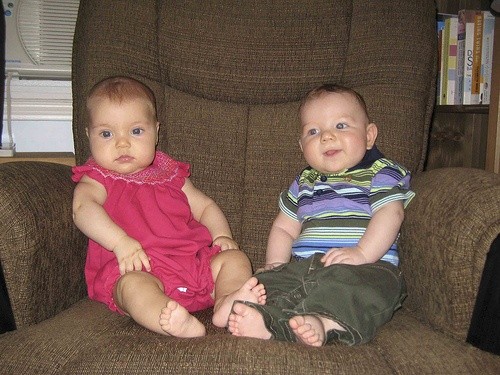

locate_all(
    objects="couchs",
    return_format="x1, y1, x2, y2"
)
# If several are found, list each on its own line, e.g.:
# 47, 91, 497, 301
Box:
0, 1, 499, 375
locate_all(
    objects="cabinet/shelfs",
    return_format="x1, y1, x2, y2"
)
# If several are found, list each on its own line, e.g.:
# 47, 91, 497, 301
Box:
422, 1, 499, 176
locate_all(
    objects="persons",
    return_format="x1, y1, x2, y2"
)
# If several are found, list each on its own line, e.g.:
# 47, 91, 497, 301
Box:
228, 86, 415, 347
72, 77, 267, 338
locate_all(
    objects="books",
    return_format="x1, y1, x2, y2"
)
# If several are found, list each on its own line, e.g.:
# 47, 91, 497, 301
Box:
437, 9, 496, 104
